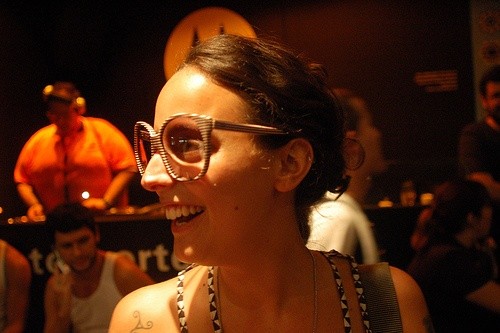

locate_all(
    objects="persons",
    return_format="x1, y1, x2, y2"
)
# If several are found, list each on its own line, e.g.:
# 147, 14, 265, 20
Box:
43, 202, 155, 333
302, 84, 389, 265
410, 66, 500, 333
106, 34, 435, 333
0, 238, 31, 333
12, 83, 136, 223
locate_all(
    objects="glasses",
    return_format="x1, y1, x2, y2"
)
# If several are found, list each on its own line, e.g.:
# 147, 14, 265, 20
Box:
134, 113, 288, 181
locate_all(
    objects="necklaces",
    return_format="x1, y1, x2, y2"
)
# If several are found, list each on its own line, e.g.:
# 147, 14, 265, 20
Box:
216, 246, 318, 333
